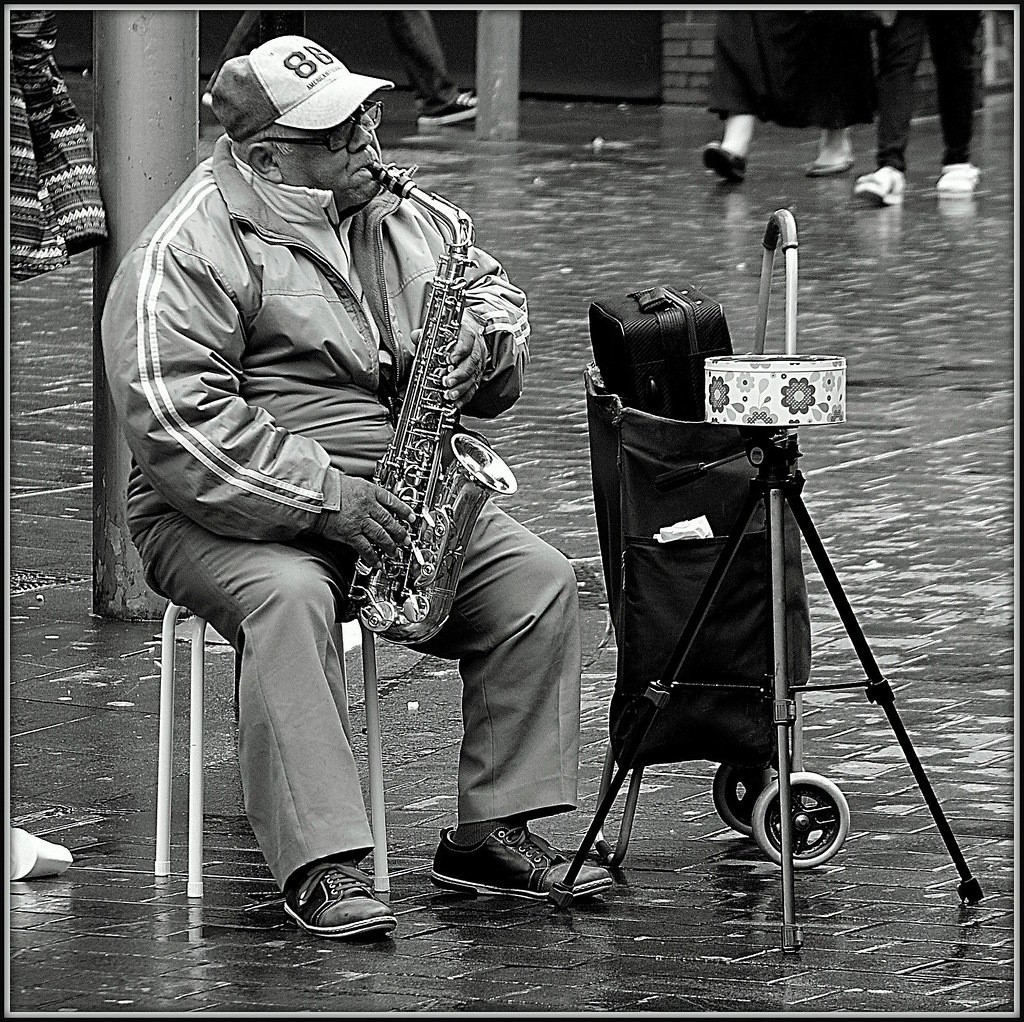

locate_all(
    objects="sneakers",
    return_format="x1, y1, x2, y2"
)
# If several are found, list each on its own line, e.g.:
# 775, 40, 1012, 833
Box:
934, 161, 982, 204
415, 90, 478, 125
854, 166, 907, 208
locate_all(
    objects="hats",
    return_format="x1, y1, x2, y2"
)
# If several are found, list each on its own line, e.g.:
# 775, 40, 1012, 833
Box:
201, 36, 398, 145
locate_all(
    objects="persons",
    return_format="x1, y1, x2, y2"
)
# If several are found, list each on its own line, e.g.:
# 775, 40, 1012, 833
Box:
202, 10, 476, 125
701, 11, 875, 185
855, 11, 986, 206
10, 11, 109, 289
102, 37, 611, 932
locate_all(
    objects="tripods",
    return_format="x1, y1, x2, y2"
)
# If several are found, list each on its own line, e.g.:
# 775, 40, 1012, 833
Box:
549, 432, 982, 950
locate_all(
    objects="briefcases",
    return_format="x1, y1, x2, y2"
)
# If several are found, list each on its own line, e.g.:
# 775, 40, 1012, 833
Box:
589, 280, 733, 421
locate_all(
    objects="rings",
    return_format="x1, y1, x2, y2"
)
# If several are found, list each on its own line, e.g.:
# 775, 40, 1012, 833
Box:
474, 383, 478, 389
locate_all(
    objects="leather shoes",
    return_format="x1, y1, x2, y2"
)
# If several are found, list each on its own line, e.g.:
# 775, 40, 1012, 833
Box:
429, 829, 613, 906
284, 861, 397, 939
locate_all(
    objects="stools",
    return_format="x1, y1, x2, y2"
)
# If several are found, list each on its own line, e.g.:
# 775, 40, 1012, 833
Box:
152, 604, 391, 900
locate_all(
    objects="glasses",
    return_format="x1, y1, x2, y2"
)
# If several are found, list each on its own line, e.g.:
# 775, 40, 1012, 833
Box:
248, 98, 383, 148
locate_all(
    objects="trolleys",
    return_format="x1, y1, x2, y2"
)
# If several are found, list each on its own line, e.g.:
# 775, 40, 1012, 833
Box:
580, 208, 850, 871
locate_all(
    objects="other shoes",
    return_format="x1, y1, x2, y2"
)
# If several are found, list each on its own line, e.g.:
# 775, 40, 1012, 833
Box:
700, 138, 747, 187
804, 157, 854, 176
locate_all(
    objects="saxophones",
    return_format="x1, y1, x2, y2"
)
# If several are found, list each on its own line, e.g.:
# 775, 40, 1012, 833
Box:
345, 160, 518, 648
